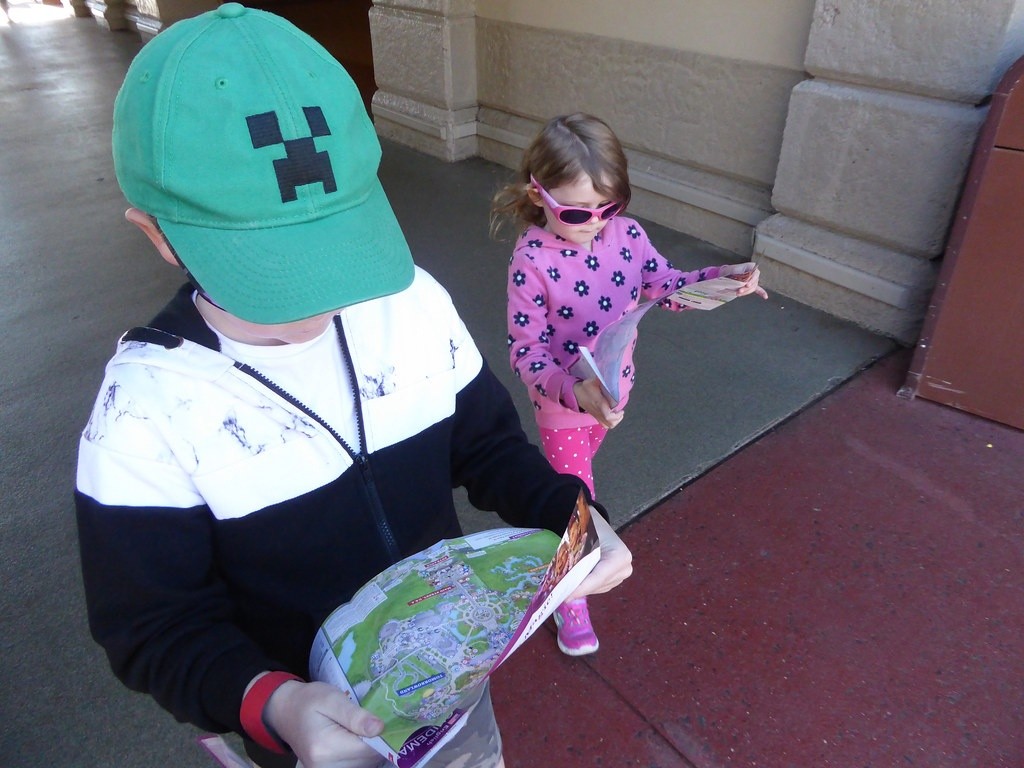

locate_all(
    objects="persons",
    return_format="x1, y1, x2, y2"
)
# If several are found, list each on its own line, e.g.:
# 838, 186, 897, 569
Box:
491, 113, 769, 657
72, 1, 634, 768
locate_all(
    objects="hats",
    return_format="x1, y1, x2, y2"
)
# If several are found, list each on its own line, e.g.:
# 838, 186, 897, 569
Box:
112, 2, 417, 326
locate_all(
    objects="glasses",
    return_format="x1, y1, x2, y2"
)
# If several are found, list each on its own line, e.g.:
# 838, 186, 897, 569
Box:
530, 172, 628, 225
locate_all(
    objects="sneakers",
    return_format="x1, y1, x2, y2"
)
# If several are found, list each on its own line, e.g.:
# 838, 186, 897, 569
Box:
553, 596, 599, 656
